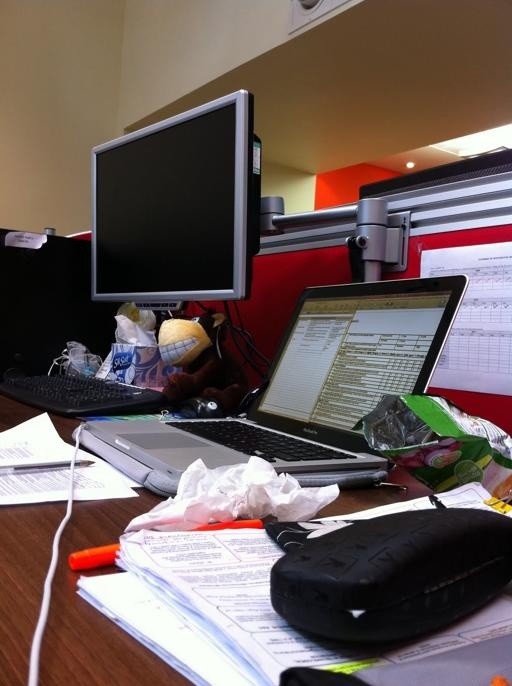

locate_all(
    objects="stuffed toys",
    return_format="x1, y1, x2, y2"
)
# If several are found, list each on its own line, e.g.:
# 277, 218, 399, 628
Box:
156, 306, 248, 411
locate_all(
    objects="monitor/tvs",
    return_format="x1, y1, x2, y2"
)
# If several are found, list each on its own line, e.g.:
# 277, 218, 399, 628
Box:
91, 89, 261, 302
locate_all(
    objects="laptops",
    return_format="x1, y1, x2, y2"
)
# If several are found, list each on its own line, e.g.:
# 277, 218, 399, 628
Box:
71, 273, 470, 498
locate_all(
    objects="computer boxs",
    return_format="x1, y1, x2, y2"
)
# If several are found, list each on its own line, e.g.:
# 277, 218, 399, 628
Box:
0, 228, 125, 382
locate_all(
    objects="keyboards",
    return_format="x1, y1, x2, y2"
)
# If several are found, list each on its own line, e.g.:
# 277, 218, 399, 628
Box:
0, 374, 167, 417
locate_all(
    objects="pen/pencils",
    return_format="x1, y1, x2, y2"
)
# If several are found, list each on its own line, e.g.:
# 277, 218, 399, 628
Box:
0, 460, 96, 476
69, 519, 264, 571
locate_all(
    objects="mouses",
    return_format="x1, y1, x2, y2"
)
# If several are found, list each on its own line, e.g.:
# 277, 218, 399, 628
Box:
174, 396, 226, 418
